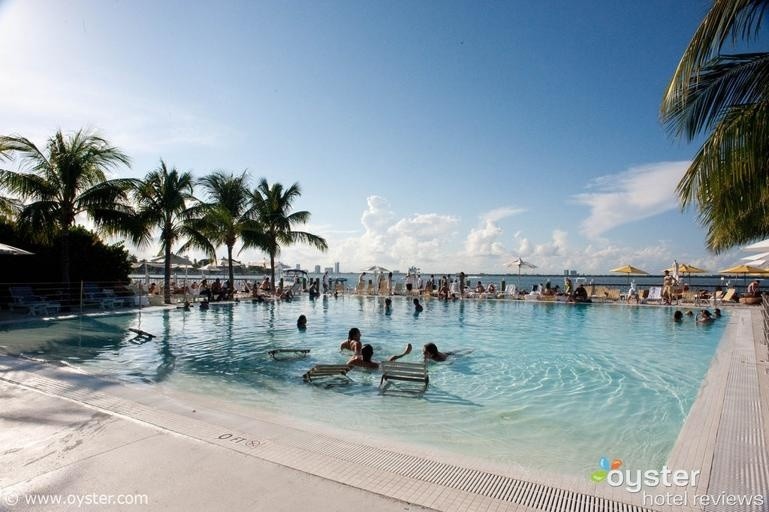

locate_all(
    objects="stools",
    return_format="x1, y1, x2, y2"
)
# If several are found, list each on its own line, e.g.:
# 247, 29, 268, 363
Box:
303, 362, 356, 390
269, 346, 312, 359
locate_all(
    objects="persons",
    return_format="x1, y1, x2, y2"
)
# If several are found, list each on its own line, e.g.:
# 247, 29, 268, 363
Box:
422, 342, 475, 363
111, 269, 760, 330
339, 327, 361, 355
345, 344, 412, 369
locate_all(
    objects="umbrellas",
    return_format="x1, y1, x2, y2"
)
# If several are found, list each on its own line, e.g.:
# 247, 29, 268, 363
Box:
609, 265, 650, 286
504, 257, 538, 295
367, 265, 387, 282
130, 251, 291, 280
663, 239, 769, 294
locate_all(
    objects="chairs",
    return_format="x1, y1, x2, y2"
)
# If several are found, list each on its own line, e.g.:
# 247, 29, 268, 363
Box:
392, 281, 736, 302
128, 328, 155, 339
379, 362, 431, 397
8, 285, 62, 317
132, 280, 302, 307
81, 283, 124, 310
